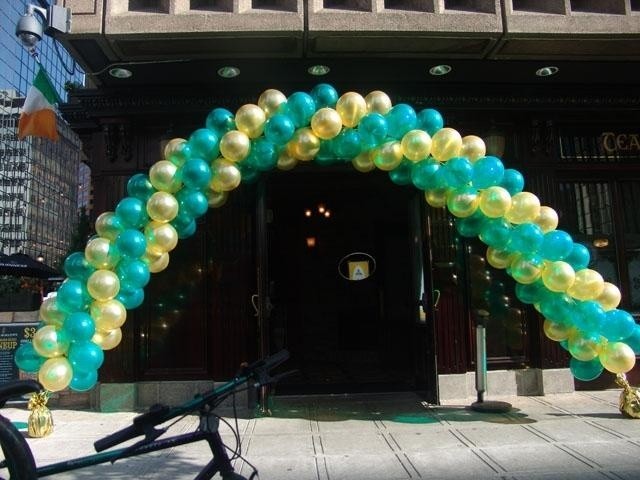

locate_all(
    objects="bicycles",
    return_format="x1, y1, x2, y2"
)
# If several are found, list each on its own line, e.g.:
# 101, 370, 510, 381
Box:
1, 349, 300, 480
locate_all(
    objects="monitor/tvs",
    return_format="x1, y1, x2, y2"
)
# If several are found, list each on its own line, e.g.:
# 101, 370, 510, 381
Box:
347, 260, 370, 280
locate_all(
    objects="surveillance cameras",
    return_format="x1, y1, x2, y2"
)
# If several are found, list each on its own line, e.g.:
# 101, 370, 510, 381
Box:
17, 14, 42, 47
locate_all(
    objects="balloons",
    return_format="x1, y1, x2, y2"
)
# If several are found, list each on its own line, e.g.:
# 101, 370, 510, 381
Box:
478, 219, 639, 380
150, 89, 294, 238
16, 173, 179, 393
276, 84, 342, 170
336, 90, 558, 237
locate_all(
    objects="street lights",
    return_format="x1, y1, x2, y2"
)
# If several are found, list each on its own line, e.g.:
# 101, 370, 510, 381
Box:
35, 252, 45, 293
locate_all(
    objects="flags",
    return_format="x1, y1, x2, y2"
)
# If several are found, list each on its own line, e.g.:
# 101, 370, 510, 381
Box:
17, 68, 62, 141
